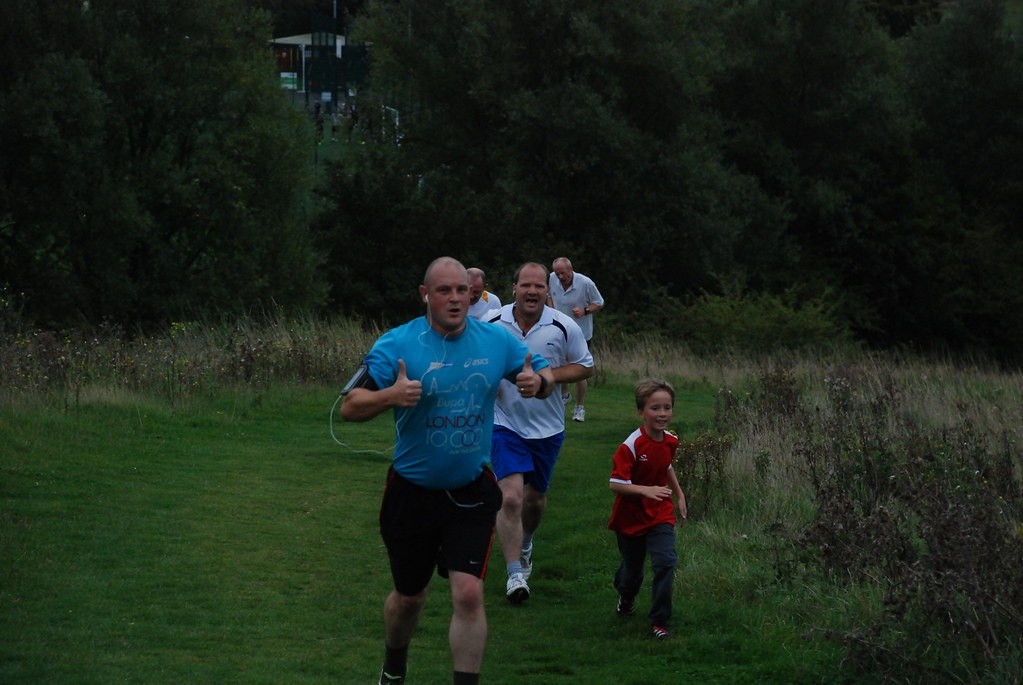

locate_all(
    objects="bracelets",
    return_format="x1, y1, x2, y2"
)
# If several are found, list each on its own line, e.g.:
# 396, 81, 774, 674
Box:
536, 373, 548, 396
584, 307, 590, 315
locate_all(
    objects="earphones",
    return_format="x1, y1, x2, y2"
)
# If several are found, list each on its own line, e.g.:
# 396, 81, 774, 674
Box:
512, 291, 516, 297
425, 294, 429, 303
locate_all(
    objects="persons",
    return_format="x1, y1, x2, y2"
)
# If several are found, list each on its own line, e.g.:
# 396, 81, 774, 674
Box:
465, 268, 502, 318
480, 262, 594, 602
547, 257, 605, 422
312, 98, 357, 130
609, 378, 688, 639
341, 257, 555, 685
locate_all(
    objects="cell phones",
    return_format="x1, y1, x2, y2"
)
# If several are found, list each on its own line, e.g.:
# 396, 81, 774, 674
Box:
339, 365, 367, 395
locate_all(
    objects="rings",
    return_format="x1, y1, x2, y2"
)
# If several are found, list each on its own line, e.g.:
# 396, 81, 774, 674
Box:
521, 388, 524, 393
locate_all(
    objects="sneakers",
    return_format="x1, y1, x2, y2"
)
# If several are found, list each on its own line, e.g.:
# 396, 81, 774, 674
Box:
617, 596, 635, 617
520, 542, 532, 580
562, 392, 572, 406
651, 623, 673, 640
505, 573, 531, 602
573, 405, 586, 422
379, 664, 407, 685
